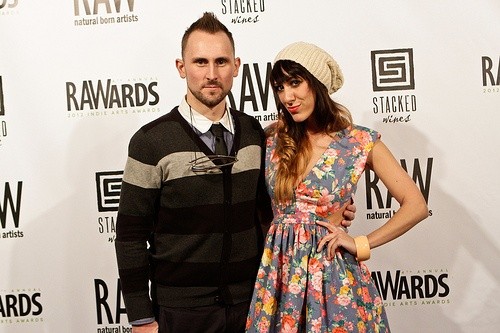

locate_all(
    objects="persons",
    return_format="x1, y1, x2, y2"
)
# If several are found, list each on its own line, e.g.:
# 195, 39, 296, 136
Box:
245, 41, 429, 333
115, 11, 356, 333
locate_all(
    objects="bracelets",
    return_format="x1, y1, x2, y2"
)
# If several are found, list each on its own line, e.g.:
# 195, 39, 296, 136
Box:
353, 236, 370, 262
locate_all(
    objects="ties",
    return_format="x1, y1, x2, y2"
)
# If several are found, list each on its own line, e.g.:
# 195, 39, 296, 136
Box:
209, 124, 230, 165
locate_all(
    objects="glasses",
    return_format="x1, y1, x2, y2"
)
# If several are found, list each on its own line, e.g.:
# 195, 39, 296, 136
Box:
189, 155, 239, 172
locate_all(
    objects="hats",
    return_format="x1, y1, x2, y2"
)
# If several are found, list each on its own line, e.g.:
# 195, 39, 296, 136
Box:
275, 42, 344, 94
